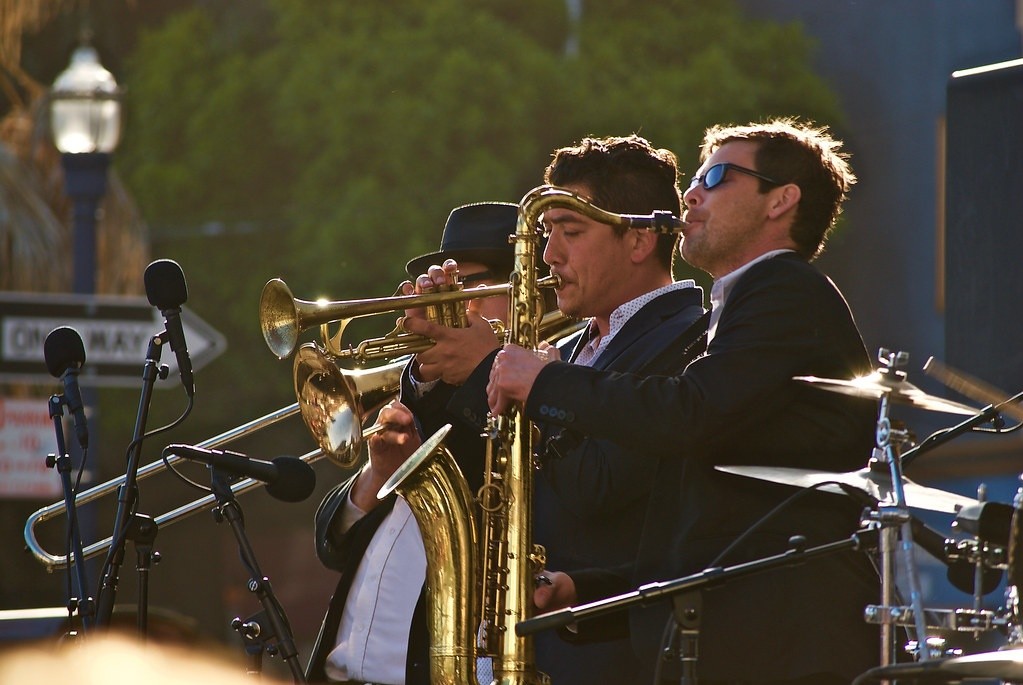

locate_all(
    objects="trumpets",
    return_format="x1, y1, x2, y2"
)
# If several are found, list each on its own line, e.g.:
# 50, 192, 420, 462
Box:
259, 268, 565, 361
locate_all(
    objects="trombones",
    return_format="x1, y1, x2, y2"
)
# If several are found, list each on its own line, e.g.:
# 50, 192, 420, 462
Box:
23, 308, 588, 568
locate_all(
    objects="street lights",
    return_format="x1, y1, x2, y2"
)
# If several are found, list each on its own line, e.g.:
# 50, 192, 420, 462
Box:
48, 42, 123, 294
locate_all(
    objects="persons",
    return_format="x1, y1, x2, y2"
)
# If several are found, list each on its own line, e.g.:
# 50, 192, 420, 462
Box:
403, 121, 710, 685
304, 200, 558, 685
485, 117, 915, 685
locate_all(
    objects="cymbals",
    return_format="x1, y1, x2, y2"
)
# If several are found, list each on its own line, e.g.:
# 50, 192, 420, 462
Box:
790, 368, 984, 417
714, 463, 981, 515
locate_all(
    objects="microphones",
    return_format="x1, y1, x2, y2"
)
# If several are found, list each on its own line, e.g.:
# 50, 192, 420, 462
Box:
840, 484, 1003, 596
144, 259, 196, 398
169, 444, 315, 503
43, 326, 89, 449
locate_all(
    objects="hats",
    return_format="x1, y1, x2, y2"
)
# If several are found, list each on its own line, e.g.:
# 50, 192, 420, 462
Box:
405, 202, 548, 278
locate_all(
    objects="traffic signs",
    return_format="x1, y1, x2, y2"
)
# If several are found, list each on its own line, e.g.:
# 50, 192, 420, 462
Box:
0, 288, 229, 393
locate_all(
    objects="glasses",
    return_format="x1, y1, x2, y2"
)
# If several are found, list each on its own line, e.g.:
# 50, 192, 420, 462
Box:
458, 270, 493, 288
689, 163, 782, 189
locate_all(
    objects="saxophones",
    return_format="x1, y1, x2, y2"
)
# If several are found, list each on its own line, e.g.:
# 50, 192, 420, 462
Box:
375, 185, 689, 685
